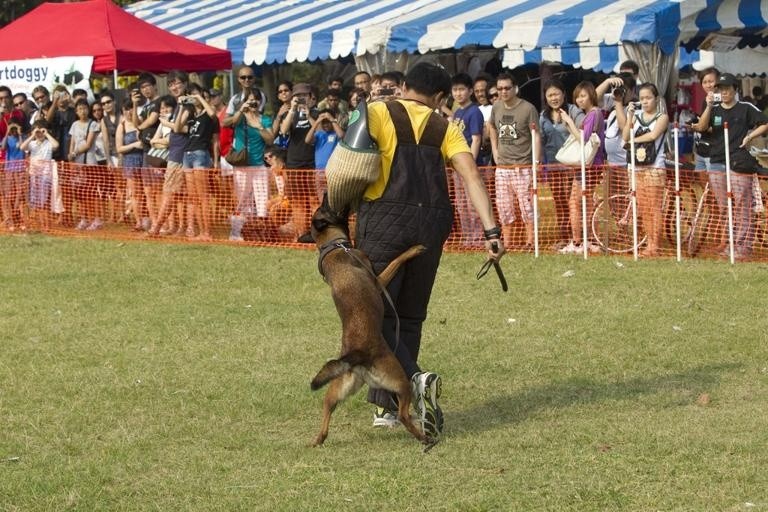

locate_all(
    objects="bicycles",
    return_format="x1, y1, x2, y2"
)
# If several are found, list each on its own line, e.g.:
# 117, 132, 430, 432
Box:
687, 140, 767, 259
589, 144, 697, 253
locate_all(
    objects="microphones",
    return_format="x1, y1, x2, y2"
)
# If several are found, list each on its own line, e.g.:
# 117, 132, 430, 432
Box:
38, 102, 43, 116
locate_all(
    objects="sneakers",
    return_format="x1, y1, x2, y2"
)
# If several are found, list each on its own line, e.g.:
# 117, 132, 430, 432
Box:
558, 241, 602, 254
120, 214, 210, 240
56, 217, 115, 233
371, 406, 399, 428
408, 372, 443, 440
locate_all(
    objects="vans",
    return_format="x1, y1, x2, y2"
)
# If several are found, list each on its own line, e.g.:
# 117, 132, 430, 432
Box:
667, 79, 705, 121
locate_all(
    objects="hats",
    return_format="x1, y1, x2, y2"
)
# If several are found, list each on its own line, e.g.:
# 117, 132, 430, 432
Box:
291, 83, 311, 97
713, 73, 737, 86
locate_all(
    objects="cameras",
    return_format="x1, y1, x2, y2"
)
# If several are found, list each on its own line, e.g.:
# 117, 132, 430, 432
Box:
712, 93, 722, 102
58, 92, 65, 96
247, 102, 258, 108
631, 102, 642, 110
182, 96, 196, 105
612, 83, 626, 98
294, 97, 306, 104
684, 112, 700, 125
378, 88, 394, 95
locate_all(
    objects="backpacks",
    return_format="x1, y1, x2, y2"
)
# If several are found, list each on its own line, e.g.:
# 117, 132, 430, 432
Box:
624, 115, 666, 164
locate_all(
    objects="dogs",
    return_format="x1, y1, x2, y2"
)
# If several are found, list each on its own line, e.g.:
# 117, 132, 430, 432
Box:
297, 192, 435, 447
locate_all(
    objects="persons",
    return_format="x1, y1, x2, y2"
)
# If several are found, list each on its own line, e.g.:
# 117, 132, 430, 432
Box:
324, 62, 506, 427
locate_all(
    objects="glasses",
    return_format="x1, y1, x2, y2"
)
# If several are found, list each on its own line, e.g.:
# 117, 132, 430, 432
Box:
240, 75, 253, 79
486, 93, 497, 98
102, 100, 112, 105
496, 86, 511, 90
278, 89, 288, 92
13, 100, 24, 107
36, 95, 45, 102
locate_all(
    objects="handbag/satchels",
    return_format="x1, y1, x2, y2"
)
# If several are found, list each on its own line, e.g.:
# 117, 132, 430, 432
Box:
224, 147, 246, 165
729, 149, 757, 174
145, 146, 169, 168
142, 128, 157, 145
554, 129, 600, 165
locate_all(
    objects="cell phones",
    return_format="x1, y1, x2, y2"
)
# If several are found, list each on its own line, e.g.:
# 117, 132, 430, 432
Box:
562, 104, 568, 114
132, 88, 140, 98
